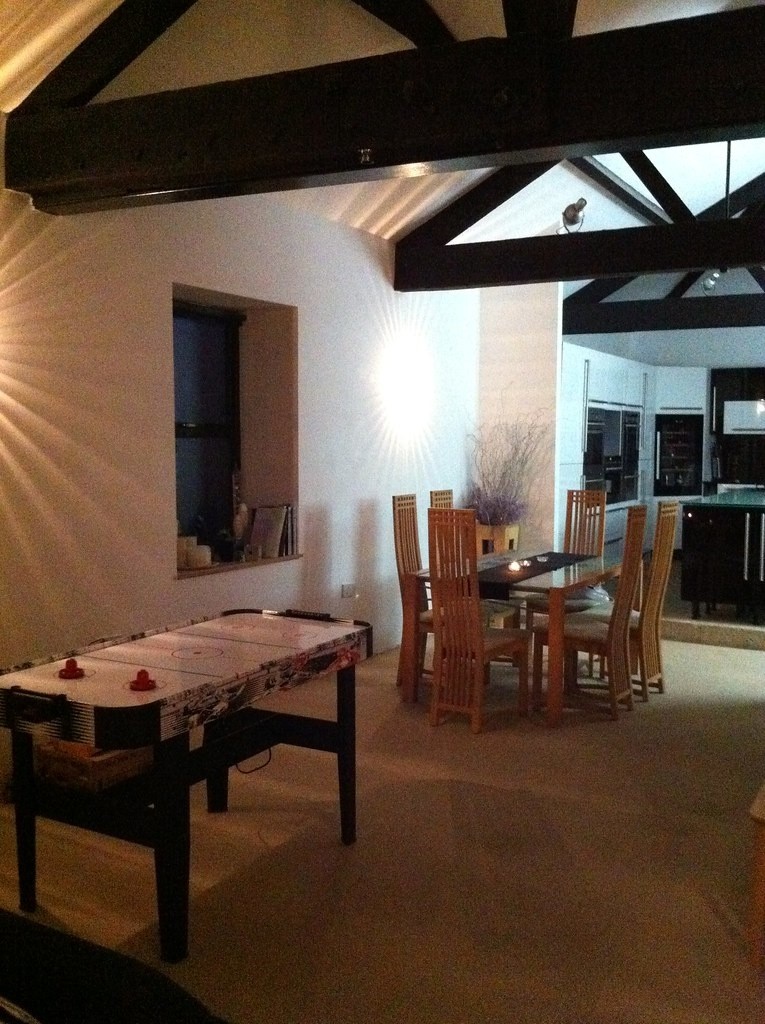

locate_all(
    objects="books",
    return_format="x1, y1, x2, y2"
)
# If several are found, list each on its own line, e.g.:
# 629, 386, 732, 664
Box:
250, 503, 296, 558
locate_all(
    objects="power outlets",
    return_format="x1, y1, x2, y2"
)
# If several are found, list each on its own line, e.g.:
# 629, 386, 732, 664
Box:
342, 582, 356, 598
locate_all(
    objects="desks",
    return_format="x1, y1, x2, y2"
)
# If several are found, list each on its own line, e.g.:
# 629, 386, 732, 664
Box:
0, 608, 374, 962
408, 549, 644, 727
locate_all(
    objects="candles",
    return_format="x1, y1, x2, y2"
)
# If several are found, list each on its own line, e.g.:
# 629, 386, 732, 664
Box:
66, 659, 77, 672
137, 670, 149, 686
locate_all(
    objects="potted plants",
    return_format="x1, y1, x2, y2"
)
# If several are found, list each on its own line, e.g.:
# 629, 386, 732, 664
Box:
462, 429, 552, 556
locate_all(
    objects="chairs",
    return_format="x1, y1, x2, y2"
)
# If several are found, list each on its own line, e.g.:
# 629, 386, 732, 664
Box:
392, 489, 680, 735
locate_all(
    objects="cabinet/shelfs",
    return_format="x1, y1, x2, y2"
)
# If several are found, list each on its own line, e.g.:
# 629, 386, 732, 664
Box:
680, 491, 765, 621
587, 499, 700, 570
